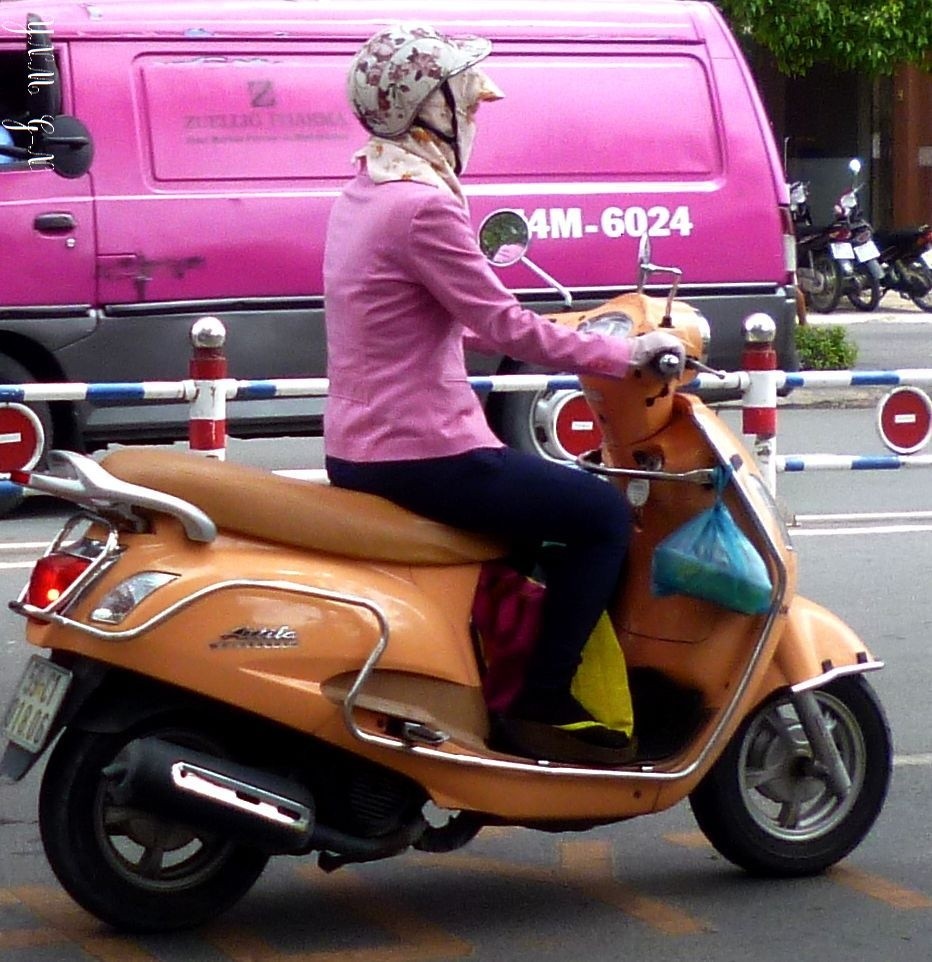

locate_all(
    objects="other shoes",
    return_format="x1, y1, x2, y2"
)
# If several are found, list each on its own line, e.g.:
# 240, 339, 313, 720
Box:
510, 683, 631, 764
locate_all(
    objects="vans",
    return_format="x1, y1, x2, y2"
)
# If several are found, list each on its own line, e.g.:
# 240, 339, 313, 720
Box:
0, 0, 809, 523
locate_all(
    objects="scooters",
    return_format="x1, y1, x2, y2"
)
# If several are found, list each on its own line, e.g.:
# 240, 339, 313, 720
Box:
0, 212, 893, 933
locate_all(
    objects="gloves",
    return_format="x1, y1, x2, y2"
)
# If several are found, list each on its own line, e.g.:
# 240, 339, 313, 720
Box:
633, 329, 687, 382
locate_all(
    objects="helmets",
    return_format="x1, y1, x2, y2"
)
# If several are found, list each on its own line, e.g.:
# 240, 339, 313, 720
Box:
344, 22, 494, 134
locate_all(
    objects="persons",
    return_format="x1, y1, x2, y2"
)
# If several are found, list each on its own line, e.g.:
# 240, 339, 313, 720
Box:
323, 21, 686, 766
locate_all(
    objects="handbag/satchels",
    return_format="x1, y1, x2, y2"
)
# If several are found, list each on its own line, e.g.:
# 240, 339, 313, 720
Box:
651, 464, 772, 615
478, 562, 548, 719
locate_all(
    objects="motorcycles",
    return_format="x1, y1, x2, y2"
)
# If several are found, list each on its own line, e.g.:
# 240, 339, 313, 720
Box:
832, 158, 932, 313
779, 135, 885, 313
